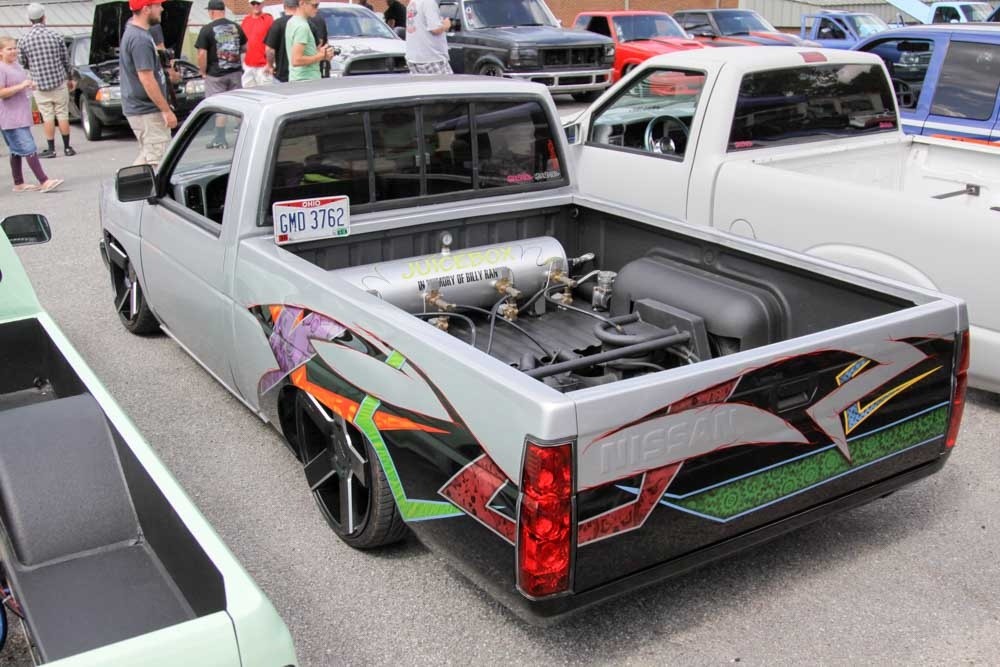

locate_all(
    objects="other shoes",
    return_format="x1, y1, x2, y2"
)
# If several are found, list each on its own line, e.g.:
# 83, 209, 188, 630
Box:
38, 149, 56, 157
65, 147, 75, 155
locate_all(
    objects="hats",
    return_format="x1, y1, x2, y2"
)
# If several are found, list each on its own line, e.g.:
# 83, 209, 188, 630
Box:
129, 0, 166, 11
249, 0, 264, 4
205, 0, 224, 11
26, 3, 44, 20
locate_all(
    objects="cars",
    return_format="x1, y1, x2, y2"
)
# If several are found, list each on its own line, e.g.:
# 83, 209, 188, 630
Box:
259, 2, 414, 79
668, 9, 823, 52
59, 0, 206, 141
571, 10, 715, 99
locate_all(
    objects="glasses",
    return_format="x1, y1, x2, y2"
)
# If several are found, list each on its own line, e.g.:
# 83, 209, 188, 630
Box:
304, 2, 318, 8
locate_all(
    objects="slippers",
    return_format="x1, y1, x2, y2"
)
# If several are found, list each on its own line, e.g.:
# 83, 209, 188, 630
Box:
40, 179, 63, 193
13, 183, 39, 192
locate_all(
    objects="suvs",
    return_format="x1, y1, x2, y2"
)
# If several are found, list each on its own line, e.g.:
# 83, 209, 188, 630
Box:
436, 0, 616, 105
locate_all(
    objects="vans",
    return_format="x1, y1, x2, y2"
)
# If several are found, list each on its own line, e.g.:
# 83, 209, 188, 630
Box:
846, 21, 1000, 145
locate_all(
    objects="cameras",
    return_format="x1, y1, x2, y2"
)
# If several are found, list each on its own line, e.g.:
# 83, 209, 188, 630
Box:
328, 46, 341, 55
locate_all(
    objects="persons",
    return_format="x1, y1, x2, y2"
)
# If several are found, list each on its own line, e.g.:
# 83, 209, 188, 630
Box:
239, 0, 274, 88
405, 0, 454, 74
17, 3, 76, 157
384, 0, 406, 27
119, 0, 178, 175
358, 0, 373, 11
264, 0, 330, 84
0, 35, 64, 192
284, 0, 334, 81
194, 0, 248, 149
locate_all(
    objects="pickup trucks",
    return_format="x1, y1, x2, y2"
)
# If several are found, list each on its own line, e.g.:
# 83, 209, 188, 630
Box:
98, 71, 974, 631
880, 0, 1000, 34
557, 44, 1000, 400
790, 10, 932, 79
